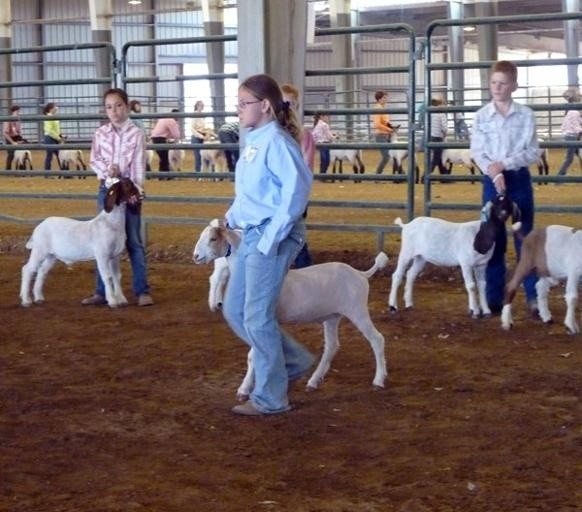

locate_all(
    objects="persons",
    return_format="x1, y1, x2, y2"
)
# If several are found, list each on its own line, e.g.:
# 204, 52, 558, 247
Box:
129, 100, 151, 172
81, 88, 154, 307
2, 105, 22, 170
280, 84, 316, 268
312, 114, 340, 174
421, 99, 448, 184
218, 122, 239, 171
191, 101, 210, 172
150, 109, 180, 180
223, 74, 314, 416
373, 92, 404, 174
470, 60, 540, 318
557, 95, 582, 175
43, 102, 63, 179
448, 101, 469, 140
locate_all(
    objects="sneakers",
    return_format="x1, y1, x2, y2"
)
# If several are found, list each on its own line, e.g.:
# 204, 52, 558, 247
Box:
137, 292, 154, 307
233, 399, 262, 419
80, 294, 106, 306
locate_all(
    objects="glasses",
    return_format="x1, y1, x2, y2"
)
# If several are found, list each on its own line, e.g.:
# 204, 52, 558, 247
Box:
233, 100, 260, 109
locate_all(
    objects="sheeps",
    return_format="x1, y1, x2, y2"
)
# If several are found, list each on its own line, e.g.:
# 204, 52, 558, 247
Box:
432, 137, 484, 185
534, 137, 550, 186
499, 221, 582, 337
55, 133, 87, 180
328, 133, 365, 184
387, 191, 524, 321
196, 127, 225, 182
165, 138, 185, 181
12, 137, 33, 177
191, 216, 246, 268
387, 122, 420, 185
144, 142, 155, 181
206, 250, 394, 405
17, 167, 147, 310
